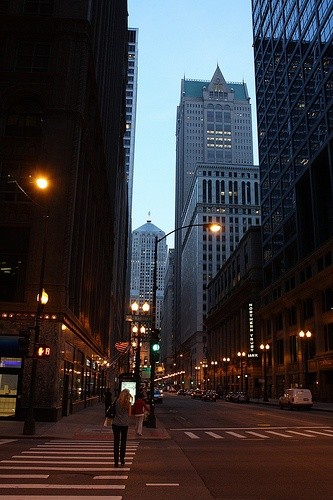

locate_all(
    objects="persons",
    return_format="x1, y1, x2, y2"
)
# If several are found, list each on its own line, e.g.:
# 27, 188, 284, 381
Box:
112, 389, 132, 467
104, 397, 112, 426
132, 394, 149, 436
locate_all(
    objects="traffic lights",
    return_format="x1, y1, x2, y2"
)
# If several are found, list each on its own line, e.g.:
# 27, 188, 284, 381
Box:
150, 341, 160, 363
34, 344, 52, 359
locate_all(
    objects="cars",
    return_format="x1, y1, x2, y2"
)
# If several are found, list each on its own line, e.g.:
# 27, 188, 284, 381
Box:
176, 388, 219, 402
225, 392, 250, 403
279, 389, 313, 410
146, 387, 164, 404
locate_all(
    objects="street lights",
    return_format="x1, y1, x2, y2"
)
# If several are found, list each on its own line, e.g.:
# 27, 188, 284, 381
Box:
237, 351, 246, 392
23, 287, 52, 435
223, 358, 230, 392
154, 371, 185, 389
144, 223, 223, 428
299, 331, 312, 388
202, 364, 208, 389
195, 366, 201, 388
259, 344, 270, 402
129, 300, 151, 400
211, 361, 218, 390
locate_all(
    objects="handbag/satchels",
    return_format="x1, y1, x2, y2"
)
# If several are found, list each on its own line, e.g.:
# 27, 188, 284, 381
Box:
105, 405, 115, 418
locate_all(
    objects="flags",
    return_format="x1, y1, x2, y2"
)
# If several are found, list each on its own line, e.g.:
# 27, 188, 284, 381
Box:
115, 342, 129, 356
